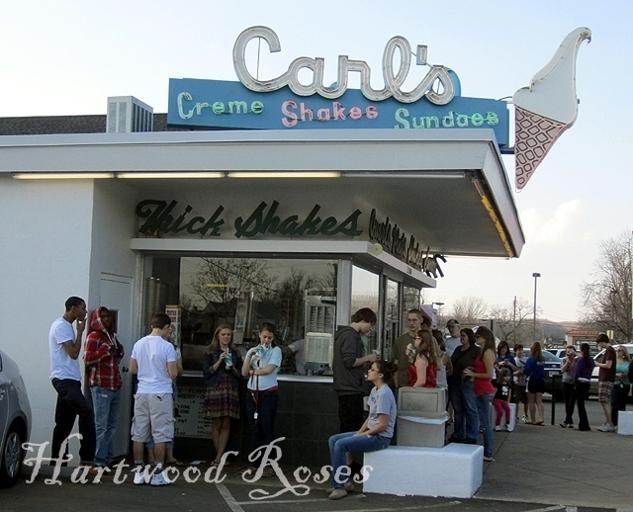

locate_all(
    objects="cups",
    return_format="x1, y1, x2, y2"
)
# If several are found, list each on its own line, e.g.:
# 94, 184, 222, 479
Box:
224, 353, 231, 366
255, 345, 265, 358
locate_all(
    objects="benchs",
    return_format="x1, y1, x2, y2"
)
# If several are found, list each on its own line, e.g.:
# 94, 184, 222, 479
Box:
362, 443, 485, 499
617, 411, 633, 436
489, 403, 517, 432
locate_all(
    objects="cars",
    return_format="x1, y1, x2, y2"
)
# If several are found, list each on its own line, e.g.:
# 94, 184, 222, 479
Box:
0, 348, 32, 482
492, 343, 633, 398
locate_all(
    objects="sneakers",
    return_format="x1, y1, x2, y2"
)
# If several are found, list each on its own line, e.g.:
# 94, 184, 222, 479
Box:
479, 414, 546, 433
559, 421, 591, 432
326, 485, 347, 500
597, 422, 615, 433
345, 481, 362, 492
132, 468, 174, 487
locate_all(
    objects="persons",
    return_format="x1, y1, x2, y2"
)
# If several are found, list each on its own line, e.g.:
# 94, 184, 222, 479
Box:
83, 304, 125, 474
241, 322, 282, 475
145, 327, 184, 467
48, 296, 96, 470
202, 324, 242, 470
127, 311, 179, 488
283, 340, 324, 375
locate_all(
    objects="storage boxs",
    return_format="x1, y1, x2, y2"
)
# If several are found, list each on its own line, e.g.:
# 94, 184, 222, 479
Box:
395, 385, 452, 448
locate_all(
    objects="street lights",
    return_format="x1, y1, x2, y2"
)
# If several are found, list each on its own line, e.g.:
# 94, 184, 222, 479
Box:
432, 302, 443, 307
531, 272, 541, 344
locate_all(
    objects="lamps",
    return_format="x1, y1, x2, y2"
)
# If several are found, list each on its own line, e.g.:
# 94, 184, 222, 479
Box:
471, 178, 516, 262
12, 170, 469, 180
372, 242, 383, 256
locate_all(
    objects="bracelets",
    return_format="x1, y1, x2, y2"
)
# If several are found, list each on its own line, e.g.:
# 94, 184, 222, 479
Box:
252, 369, 255, 375
